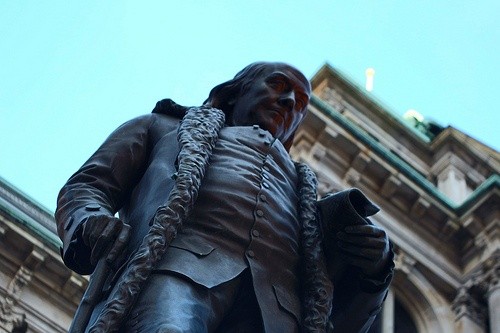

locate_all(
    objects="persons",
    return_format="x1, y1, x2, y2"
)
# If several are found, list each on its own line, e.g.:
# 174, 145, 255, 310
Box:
54, 60, 397, 333
